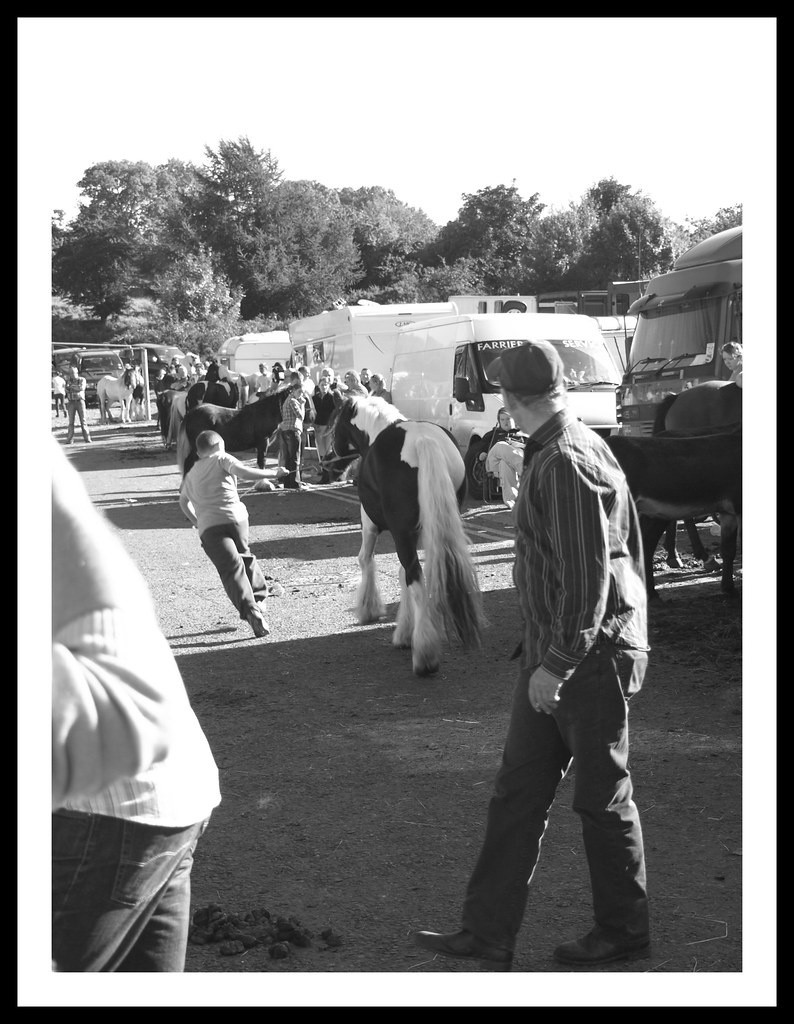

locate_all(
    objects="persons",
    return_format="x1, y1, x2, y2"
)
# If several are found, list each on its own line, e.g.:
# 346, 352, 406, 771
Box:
721, 341, 742, 389
178, 430, 272, 637
50, 434, 221, 971
51, 351, 393, 490
477, 407, 529, 510
417, 339, 653, 972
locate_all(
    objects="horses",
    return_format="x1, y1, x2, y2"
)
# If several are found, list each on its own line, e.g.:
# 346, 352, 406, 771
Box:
157, 360, 318, 493
96, 364, 145, 426
605, 380, 742, 608
327, 387, 493, 676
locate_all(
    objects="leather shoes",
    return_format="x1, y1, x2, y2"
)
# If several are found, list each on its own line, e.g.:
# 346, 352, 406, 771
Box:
412, 929, 512, 971
553, 929, 650, 966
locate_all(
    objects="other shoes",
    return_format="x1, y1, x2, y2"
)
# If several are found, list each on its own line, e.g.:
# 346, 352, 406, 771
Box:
246, 598, 269, 638
85, 439, 92, 443
56, 414, 59, 417
65, 439, 74, 444
64, 411, 68, 418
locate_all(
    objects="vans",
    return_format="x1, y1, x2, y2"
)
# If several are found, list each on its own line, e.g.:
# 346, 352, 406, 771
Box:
51, 303, 631, 502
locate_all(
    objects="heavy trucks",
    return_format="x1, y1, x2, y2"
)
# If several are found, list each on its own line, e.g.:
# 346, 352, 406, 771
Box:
614, 222, 743, 529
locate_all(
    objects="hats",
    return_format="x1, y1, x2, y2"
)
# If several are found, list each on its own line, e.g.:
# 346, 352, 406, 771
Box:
486, 340, 564, 396
